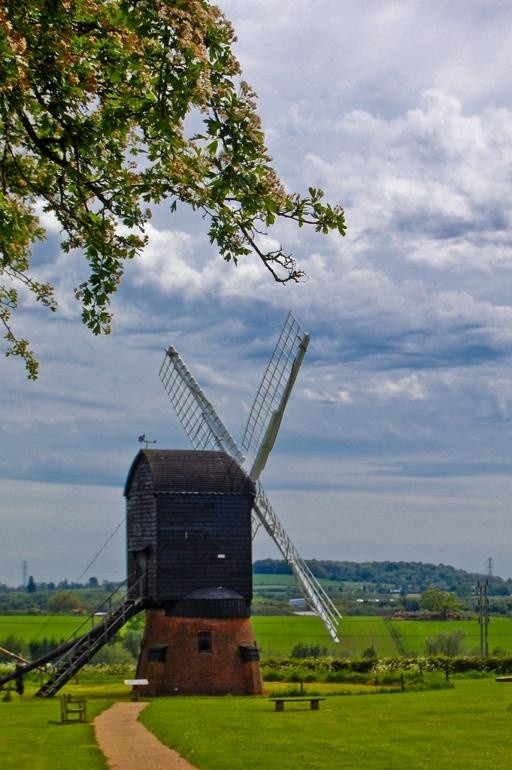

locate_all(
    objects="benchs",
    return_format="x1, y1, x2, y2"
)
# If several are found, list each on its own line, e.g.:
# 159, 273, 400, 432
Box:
268, 698, 326, 711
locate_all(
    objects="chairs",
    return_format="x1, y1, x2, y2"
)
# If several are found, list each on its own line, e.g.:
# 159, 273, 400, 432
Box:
59, 693, 86, 724
